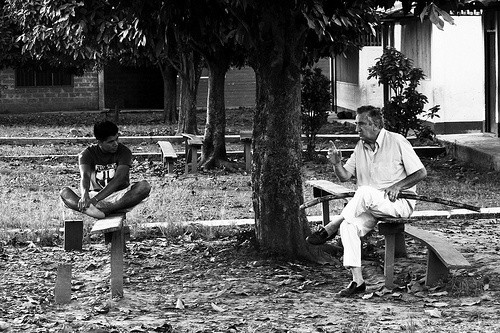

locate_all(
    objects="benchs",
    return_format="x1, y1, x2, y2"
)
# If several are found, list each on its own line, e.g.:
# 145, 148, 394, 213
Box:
159, 140, 178, 173
306, 179, 419, 288
181, 133, 203, 172
91, 212, 126, 299
402, 225, 472, 287
240, 130, 253, 172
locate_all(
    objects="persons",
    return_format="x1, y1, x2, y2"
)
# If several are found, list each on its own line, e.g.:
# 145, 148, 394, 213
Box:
305, 106, 427, 298
60, 120, 152, 219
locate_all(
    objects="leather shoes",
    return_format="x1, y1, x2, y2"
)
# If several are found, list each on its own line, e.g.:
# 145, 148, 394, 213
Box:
339, 282, 366, 296
306, 226, 338, 245
